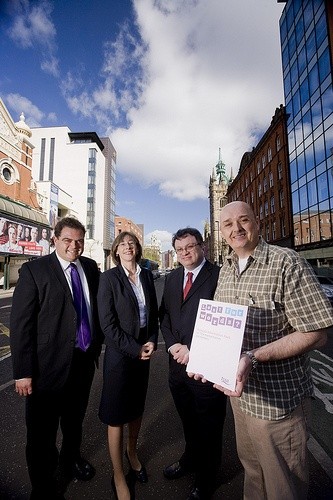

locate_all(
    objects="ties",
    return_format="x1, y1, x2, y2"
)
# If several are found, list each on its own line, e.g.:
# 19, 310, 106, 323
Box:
183, 272, 194, 299
70, 263, 93, 352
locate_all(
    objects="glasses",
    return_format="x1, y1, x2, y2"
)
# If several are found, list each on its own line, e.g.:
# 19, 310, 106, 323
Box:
175, 243, 199, 253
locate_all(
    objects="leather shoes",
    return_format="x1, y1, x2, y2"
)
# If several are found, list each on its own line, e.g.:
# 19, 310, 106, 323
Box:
60, 449, 94, 481
187, 481, 211, 500
163, 460, 194, 480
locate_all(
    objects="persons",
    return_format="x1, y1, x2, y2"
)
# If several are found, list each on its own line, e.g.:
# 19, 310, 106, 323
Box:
0, 218, 50, 257
158, 228, 228, 500
96, 231, 159, 500
186, 200, 333, 500
9, 217, 103, 500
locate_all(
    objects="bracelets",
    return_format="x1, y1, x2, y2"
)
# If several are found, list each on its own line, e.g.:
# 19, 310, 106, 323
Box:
242, 350, 261, 372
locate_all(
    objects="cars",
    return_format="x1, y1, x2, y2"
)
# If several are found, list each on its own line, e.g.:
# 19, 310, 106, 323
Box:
316, 274, 333, 302
152, 265, 180, 280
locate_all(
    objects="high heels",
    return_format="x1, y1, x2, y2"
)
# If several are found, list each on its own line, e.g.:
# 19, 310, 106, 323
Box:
111, 473, 131, 500
125, 448, 148, 483
